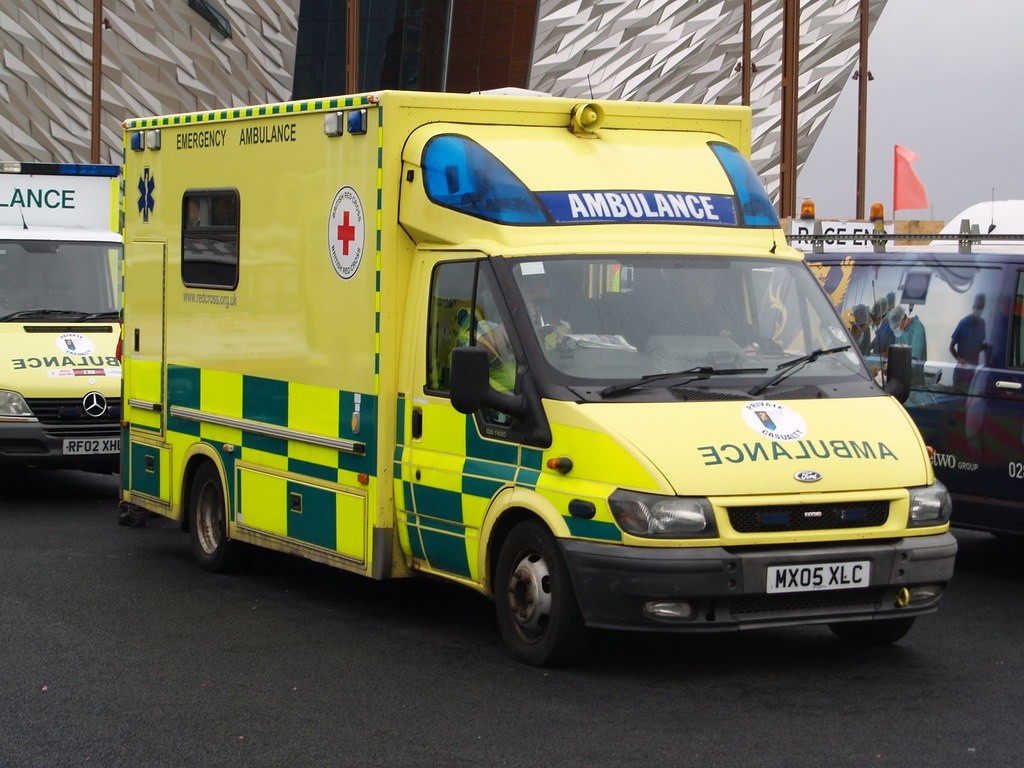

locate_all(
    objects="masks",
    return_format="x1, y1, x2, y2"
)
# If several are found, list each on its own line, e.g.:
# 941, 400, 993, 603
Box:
855, 331, 864, 346
973, 308, 983, 317
893, 328, 903, 338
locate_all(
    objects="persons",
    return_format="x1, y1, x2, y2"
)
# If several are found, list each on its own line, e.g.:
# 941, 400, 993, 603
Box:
871, 292, 897, 354
446, 258, 573, 396
861, 297, 889, 357
651, 262, 786, 357
189, 197, 208, 226
950, 291, 991, 390
883, 305, 928, 373
848, 305, 880, 377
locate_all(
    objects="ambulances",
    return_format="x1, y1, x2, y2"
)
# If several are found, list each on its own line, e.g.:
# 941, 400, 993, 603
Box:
123, 89, 958, 667
0, 162, 125, 491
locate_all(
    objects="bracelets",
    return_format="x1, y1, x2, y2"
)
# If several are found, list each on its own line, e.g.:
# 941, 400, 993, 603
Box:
753, 342, 763, 355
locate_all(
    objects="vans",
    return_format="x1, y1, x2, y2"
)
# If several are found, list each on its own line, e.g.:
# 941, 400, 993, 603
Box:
800, 250, 1024, 565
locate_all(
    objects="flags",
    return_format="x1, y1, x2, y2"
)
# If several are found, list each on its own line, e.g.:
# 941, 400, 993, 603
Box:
894, 142, 931, 210
